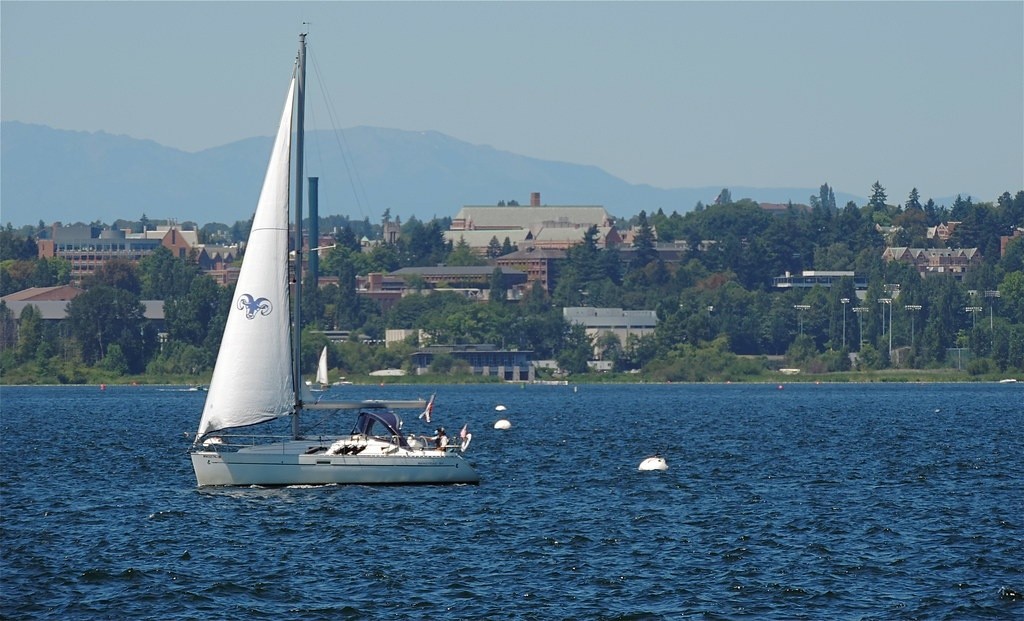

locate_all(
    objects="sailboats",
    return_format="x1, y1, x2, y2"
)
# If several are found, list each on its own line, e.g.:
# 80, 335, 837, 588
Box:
185, 29, 480, 485
310, 345, 332, 392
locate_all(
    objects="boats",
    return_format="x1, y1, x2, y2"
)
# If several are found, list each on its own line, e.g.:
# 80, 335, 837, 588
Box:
301, 397, 427, 410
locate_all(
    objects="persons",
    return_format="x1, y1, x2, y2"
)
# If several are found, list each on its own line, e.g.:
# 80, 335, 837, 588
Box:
426, 428, 450, 451
